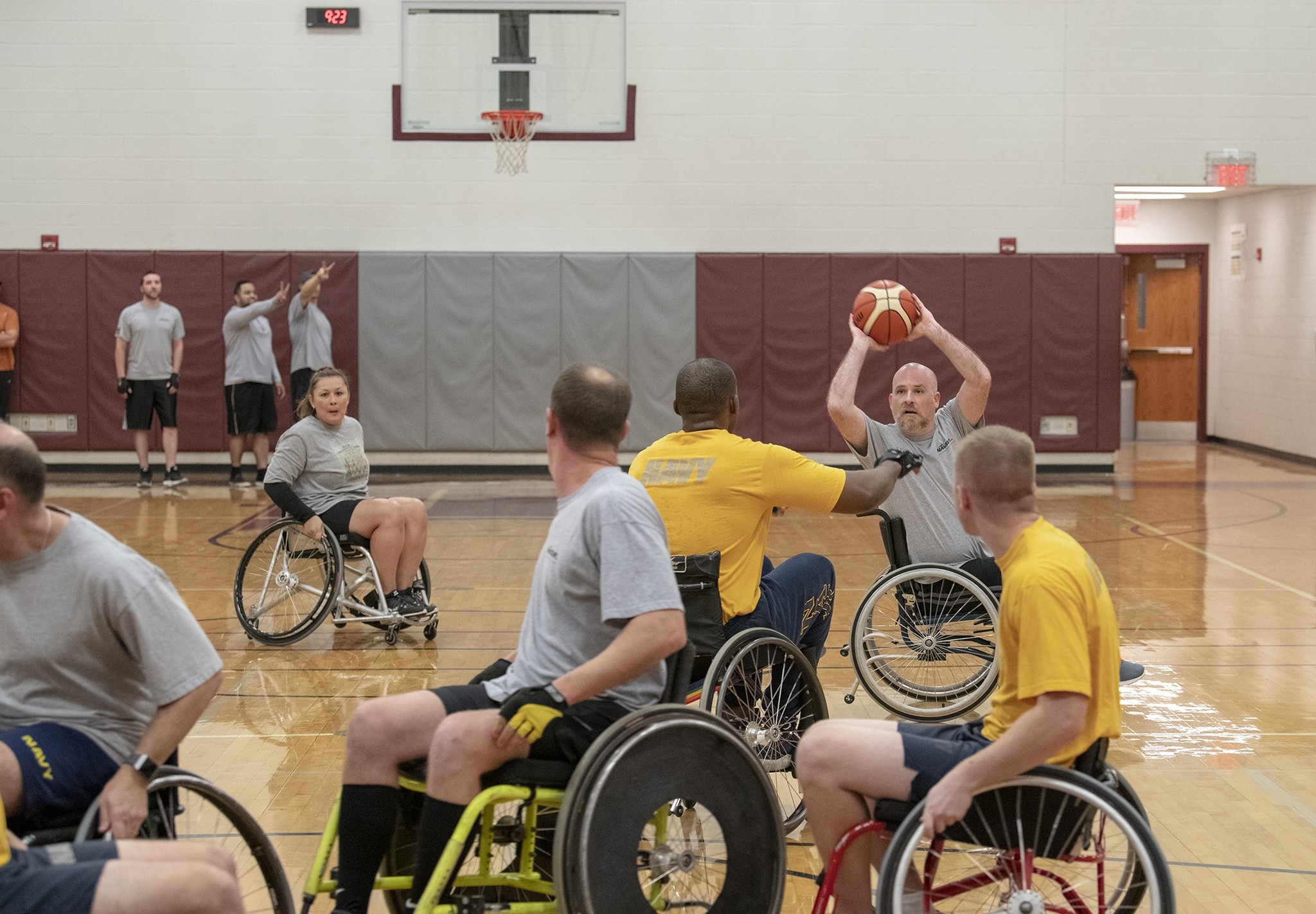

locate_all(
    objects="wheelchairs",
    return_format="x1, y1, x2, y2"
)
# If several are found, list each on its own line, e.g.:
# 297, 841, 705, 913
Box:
233, 515, 439, 647
669, 550, 831, 837
5, 763, 296, 914
811, 738, 1176, 913
296, 639, 790, 914
839, 507, 1001, 725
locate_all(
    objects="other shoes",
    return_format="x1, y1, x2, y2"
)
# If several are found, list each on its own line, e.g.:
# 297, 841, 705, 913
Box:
752, 745, 793, 773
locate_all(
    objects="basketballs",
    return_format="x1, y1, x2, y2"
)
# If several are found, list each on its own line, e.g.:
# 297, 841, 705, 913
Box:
852, 279, 916, 345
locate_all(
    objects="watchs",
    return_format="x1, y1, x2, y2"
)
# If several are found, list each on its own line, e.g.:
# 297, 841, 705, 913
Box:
122, 752, 162, 783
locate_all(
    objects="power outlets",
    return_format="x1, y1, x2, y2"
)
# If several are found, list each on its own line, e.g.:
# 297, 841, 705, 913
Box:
22, 416, 75, 429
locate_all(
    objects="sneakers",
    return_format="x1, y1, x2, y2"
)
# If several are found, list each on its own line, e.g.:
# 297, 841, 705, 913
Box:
256, 487, 269, 501
228, 473, 251, 487
162, 465, 188, 486
136, 467, 153, 487
139, 489, 153, 503
228, 488, 247, 502
162, 489, 189, 507
255, 467, 269, 486
375, 591, 428, 626
406, 588, 438, 617
1117, 658, 1146, 687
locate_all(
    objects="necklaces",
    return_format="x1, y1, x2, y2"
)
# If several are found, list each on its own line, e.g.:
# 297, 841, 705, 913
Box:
39, 508, 52, 550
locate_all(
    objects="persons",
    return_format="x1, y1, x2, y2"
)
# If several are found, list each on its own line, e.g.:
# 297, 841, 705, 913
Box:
264, 366, 438, 617
0, 422, 224, 840
0, 794, 245, 914
221, 280, 291, 487
627, 358, 924, 773
0, 302, 20, 424
114, 270, 188, 489
287, 260, 336, 424
796, 425, 1123, 914
329, 361, 689, 914
827, 293, 1146, 686
